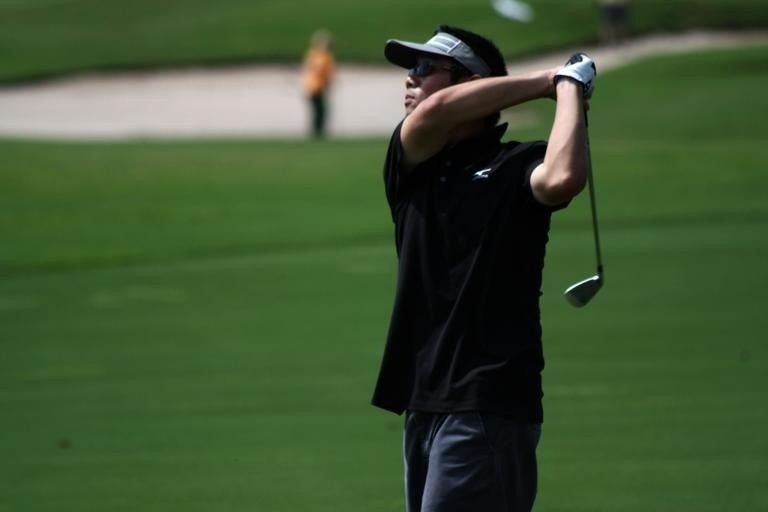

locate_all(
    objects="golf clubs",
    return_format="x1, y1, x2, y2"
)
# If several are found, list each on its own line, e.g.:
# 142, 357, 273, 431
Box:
563, 54, 604, 308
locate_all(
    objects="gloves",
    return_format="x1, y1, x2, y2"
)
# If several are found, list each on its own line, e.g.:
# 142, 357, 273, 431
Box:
551, 52, 597, 98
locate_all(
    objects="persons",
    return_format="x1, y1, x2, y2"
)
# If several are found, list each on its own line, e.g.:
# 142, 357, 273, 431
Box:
298, 27, 336, 138
383, 25, 608, 510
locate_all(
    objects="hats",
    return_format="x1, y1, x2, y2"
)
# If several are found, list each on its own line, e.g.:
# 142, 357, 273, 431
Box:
384, 32, 492, 78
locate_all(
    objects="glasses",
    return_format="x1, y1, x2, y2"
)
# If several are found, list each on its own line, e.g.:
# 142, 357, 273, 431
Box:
407, 63, 456, 78
